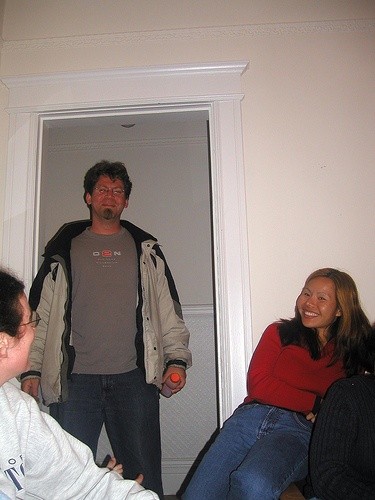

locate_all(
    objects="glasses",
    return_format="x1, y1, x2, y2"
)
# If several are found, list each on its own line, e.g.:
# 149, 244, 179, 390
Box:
19, 311, 40, 327
94, 185, 127, 197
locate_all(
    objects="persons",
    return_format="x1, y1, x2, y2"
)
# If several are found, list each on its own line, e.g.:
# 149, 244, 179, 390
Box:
20, 161, 193, 500
0, 269, 161, 500
178, 268, 375, 500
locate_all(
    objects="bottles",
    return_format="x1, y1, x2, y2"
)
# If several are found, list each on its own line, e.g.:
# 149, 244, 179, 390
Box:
161, 373, 181, 398
